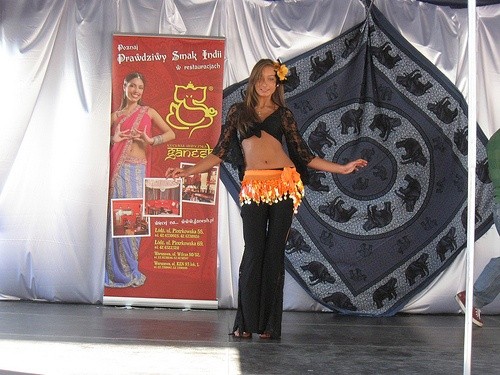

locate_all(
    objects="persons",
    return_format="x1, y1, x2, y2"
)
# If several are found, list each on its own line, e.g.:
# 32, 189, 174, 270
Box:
113, 169, 214, 238
455, 127, 500, 327
104, 71, 177, 288
166, 58, 368, 341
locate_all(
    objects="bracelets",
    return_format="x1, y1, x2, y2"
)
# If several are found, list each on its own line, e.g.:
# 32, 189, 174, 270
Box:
151, 134, 162, 147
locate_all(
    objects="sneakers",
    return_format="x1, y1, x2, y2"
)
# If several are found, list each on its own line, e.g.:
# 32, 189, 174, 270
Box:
455, 291, 485, 326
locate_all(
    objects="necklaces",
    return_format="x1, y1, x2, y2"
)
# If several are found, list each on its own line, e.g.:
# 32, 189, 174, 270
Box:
254, 105, 271, 117
